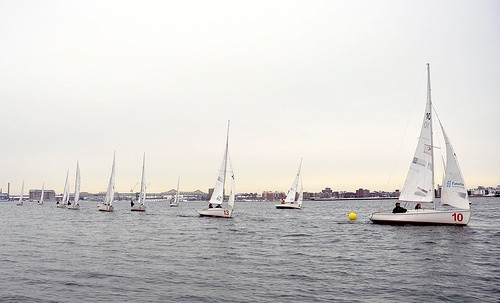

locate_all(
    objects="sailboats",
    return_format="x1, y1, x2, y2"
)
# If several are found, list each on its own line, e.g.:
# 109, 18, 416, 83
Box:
67, 161, 82, 209
170, 177, 181, 207
98, 149, 118, 211
38, 182, 45, 204
196, 119, 238, 218
130, 151, 149, 211
57, 169, 72, 208
276, 156, 304, 209
16, 181, 24, 205
369, 62, 473, 225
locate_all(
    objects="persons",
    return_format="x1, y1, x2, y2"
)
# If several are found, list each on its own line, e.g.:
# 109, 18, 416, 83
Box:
393, 202, 407, 214
415, 203, 421, 209
